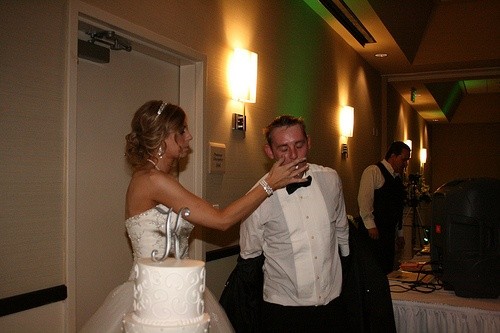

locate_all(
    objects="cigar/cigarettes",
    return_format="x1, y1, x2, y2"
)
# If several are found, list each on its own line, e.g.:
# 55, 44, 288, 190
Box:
296, 164, 298, 169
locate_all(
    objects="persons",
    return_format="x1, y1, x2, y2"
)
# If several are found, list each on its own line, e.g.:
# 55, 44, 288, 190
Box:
357, 142, 411, 273
238, 114, 353, 333
79, 99, 310, 333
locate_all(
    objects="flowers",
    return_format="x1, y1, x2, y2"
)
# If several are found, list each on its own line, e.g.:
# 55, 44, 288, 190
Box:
404, 175, 430, 201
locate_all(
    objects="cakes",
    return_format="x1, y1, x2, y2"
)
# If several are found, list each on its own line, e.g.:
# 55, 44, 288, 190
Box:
123, 256, 210, 333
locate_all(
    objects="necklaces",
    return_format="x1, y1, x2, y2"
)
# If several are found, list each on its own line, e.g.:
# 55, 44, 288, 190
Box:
148, 159, 160, 171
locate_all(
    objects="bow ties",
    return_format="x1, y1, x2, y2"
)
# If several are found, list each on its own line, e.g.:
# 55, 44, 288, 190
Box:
286, 175, 312, 195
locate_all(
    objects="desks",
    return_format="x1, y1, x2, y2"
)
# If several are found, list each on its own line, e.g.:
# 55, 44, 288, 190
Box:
387, 253, 500, 333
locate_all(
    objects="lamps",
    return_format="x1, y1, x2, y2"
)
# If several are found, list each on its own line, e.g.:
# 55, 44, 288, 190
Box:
226, 48, 259, 133
340, 106, 354, 161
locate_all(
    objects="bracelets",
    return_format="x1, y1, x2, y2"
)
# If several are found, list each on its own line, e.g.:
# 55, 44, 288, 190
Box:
260, 179, 273, 197
